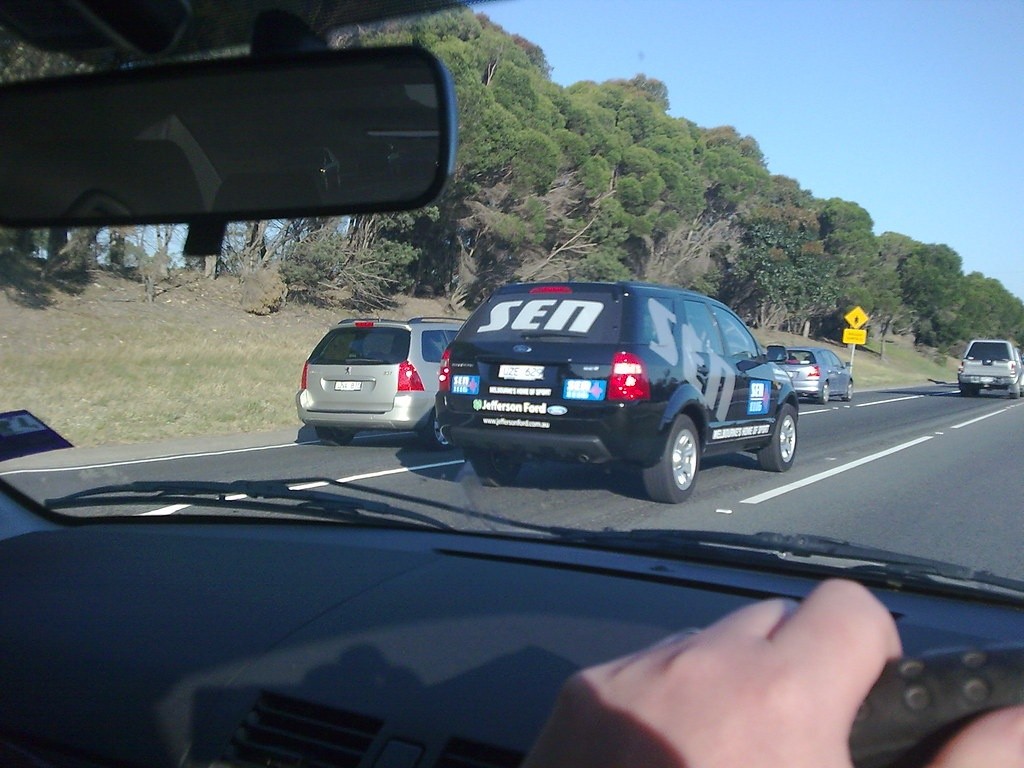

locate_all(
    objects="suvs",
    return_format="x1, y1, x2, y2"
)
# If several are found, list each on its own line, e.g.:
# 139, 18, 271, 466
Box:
441, 280, 803, 503
294, 313, 468, 447
958, 338, 1024, 398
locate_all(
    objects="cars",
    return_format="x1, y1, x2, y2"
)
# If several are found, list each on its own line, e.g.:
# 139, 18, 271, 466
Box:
779, 347, 857, 404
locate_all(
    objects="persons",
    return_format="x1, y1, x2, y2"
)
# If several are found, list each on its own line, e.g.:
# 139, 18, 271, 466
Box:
522, 578, 1024, 768
